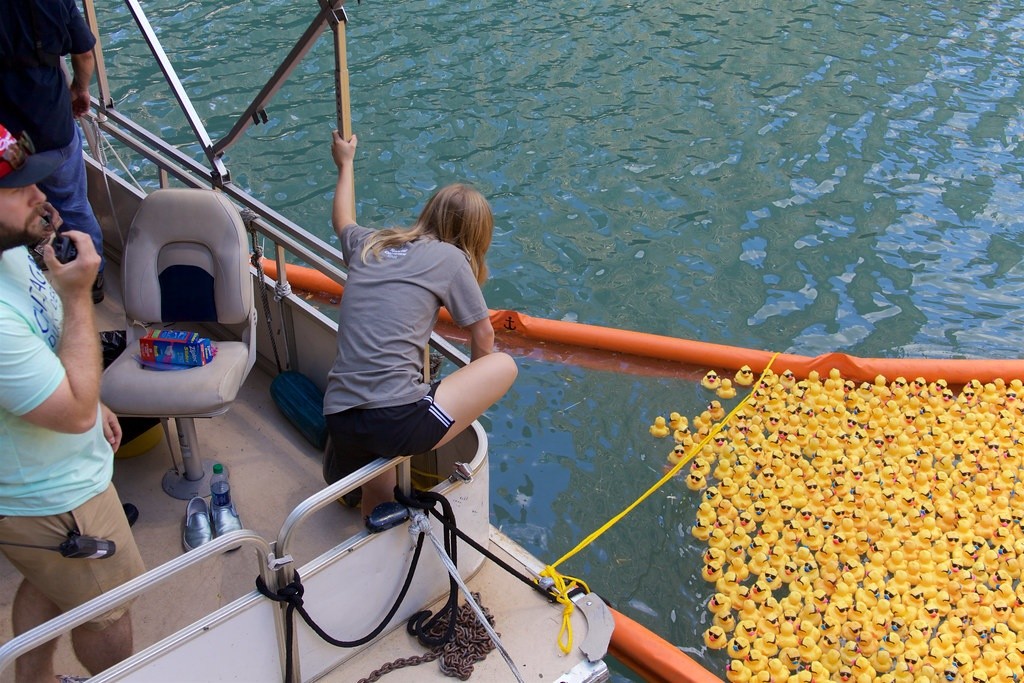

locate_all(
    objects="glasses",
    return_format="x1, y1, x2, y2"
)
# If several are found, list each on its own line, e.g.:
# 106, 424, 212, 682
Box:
0, 130, 36, 172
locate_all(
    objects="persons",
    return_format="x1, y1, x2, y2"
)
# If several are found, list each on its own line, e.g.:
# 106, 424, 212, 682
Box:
0, 125, 147, 682
0, 0, 109, 305
321, 131, 518, 528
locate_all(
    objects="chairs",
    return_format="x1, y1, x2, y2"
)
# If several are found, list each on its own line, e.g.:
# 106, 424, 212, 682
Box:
97, 188, 258, 500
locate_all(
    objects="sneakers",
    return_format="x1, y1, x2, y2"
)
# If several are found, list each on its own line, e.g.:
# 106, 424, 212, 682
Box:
122, 502, 139, 528
210, 496, 243, 551
183, 495, 213, 553
40, 264, 47, 270
91, 273, 105, 304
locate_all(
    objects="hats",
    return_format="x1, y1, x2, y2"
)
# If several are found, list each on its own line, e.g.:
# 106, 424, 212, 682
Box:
0, 124, 64, 189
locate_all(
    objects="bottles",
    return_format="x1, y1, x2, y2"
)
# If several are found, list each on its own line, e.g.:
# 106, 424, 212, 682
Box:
210, 462, 234, 519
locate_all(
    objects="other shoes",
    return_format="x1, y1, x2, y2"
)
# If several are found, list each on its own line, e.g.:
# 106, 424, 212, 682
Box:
342, 486, 362, 508
56, 673, 90, 683
